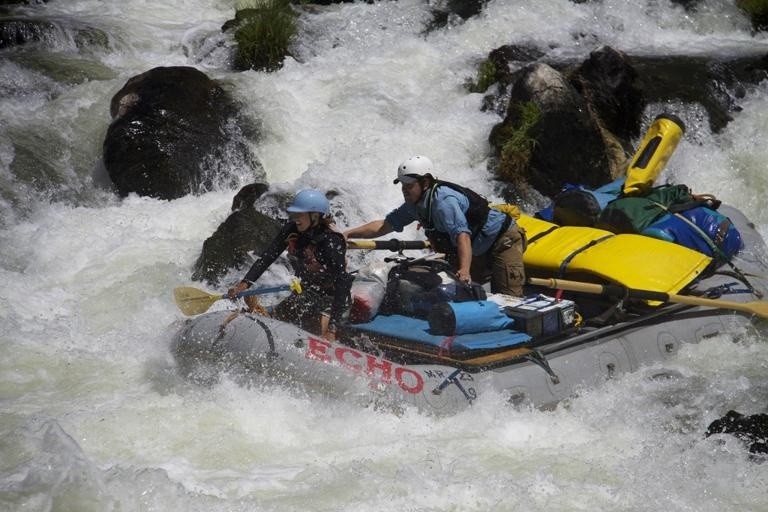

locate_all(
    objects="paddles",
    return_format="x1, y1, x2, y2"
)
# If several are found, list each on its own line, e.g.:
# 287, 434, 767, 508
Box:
529, 277, 768, 319
173, 276, 302, 316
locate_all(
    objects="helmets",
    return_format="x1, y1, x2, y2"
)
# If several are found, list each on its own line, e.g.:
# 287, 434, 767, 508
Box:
286, 189, 331, 219
393, 156, 434, 186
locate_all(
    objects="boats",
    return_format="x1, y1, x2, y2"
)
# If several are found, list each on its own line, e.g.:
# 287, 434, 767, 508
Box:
169, 180, 768, 420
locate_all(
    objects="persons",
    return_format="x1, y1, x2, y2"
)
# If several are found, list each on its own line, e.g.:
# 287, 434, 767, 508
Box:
228, 190, 352, 341
342, 155, 528, 298
